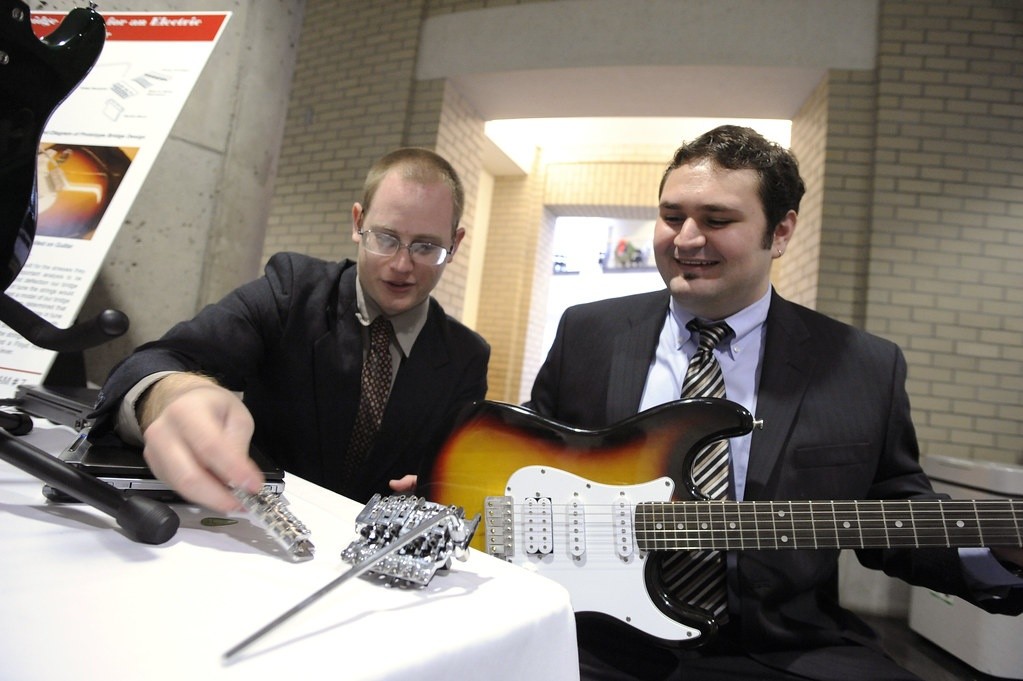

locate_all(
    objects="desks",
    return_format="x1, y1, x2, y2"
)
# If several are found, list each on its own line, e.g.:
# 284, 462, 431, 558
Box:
0, 419, 581, 681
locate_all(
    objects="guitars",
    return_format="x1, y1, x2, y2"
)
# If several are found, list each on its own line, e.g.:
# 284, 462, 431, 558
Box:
419, 392, 1023, 646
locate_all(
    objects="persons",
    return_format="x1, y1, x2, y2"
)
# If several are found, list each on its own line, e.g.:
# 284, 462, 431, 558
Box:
87, 144, 492, 516
518, 123, 1023, 681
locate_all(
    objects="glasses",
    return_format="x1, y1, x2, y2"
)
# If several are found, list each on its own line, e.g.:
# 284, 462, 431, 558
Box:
358, 225, 455, 265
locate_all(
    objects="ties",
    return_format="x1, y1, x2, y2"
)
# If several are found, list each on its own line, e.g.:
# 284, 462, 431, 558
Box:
338, 318, 394, 494
679, 319, 734, 625
